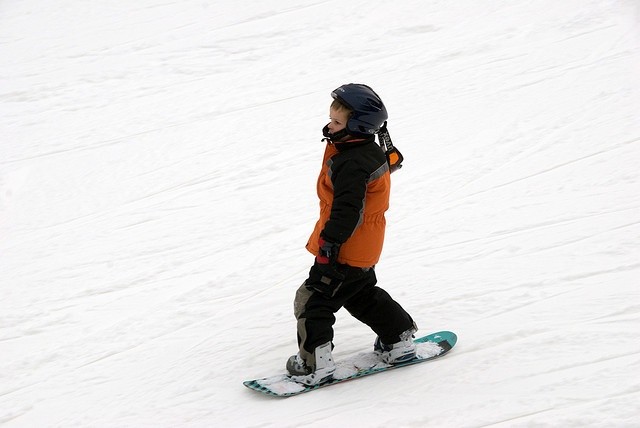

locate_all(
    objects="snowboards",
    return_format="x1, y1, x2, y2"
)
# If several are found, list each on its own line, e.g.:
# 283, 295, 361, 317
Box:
241, 330, 458, 398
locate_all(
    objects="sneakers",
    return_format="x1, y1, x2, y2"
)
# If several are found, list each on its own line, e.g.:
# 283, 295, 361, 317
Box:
286, 354, 314, 379
373, 335, 394, 353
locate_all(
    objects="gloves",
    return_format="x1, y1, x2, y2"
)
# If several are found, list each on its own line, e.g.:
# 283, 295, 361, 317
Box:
317, 235, 347, 279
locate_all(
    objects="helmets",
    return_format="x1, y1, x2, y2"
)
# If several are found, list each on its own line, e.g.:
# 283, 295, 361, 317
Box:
331, 83, 387, 134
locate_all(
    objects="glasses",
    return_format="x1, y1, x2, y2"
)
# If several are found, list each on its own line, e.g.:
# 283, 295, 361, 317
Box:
378, 126, 403, 174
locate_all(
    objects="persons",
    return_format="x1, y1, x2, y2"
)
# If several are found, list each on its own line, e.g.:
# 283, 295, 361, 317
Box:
286, 84, 418, 385
